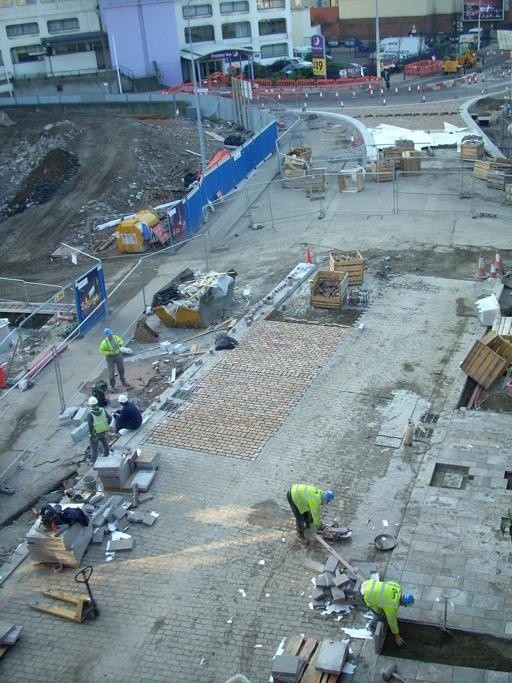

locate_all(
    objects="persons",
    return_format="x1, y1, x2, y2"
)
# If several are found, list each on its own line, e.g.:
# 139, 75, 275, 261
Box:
383, 68, 391, 91
360, 579, 415, 649
286, 483, 335, 546
86, 395, 113, 467
112, 394, 144, 437
97, 327, 132, 394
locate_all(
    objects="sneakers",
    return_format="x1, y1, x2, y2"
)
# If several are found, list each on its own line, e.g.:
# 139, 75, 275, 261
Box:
297, 536, 309, 545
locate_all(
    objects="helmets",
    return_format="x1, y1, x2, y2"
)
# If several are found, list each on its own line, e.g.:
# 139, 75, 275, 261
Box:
118, 394, 128, 402
103, 328, 112, 336
88, 396, 98, 404
326, 491, 335, 504
402, 592, 415, 606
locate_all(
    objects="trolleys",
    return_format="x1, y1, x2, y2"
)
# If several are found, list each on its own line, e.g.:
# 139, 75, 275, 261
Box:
28, 565, 101, 625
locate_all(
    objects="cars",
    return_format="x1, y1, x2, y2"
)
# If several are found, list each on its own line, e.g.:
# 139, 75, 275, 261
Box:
271, 37, 367, 79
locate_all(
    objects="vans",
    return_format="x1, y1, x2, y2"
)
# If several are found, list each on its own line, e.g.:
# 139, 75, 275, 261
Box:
368, 35, 427, 60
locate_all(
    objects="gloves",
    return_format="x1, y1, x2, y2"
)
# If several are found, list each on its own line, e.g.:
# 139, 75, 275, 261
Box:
396, 636, 405, 647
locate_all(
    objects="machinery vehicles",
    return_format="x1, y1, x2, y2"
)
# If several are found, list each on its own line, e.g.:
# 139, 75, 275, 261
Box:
442, 39, 478, 78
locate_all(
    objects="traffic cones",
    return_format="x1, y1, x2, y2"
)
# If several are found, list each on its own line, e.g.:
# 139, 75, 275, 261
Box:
475, 249, 506, 280
304, 247, 312, 264
